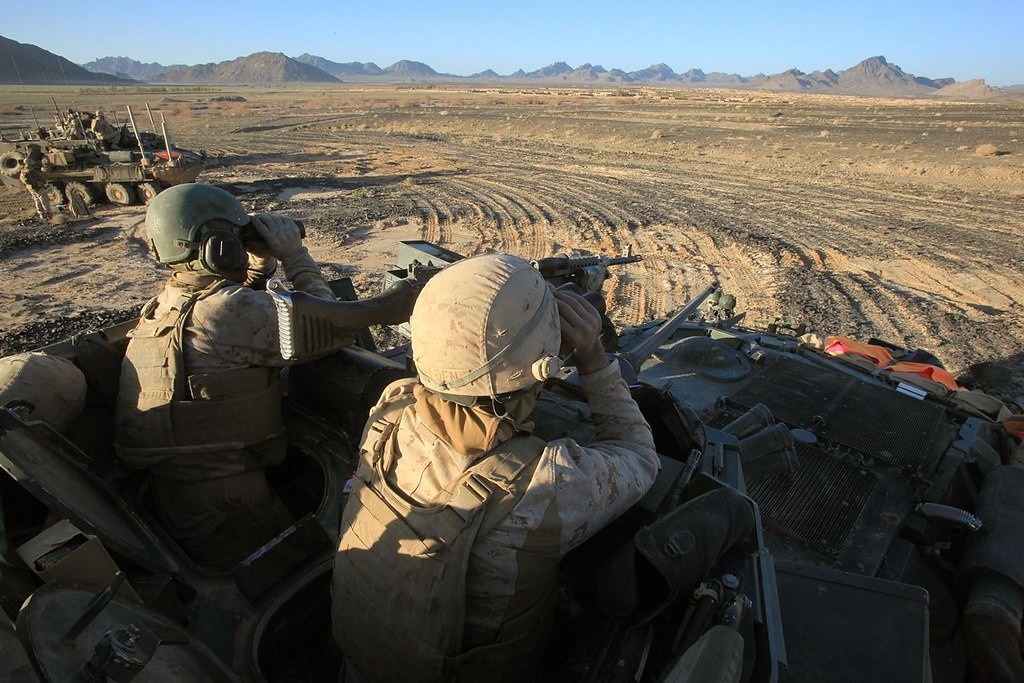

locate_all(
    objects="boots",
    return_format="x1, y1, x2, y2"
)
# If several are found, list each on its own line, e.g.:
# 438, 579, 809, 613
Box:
40, 213, 47, 219
47, 212, 52, 219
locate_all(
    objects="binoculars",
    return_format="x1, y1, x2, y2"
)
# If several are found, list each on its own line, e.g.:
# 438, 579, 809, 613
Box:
553, 281, 606, 358
241, 218, 305, 251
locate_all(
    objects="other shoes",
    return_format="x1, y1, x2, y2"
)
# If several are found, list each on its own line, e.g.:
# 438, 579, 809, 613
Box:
111, 144, 121, 150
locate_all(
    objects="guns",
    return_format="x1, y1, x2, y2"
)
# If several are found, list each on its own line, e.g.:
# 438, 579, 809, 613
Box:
574, 449, 702, 682
265, 242, 642, 362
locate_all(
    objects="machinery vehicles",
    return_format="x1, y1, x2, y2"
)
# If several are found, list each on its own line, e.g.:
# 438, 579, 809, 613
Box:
0, 239, 1024, 683
1, 111, 208, 207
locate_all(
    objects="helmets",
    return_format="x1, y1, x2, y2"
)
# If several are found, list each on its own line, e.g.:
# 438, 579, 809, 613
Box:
70, 119, 79, 127
67, 109, 73, 113
145, 184, 246, 264
96, 111, 103, 117
409, 254, 560, 396
23, 158, 35, 167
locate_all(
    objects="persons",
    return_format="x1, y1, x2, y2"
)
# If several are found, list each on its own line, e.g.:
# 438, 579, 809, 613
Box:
20, 158, 52, 220
65, 119, 83, 140
330, 253, 661, 683
92, 110, 120, 149
113, 183, 337, 572
